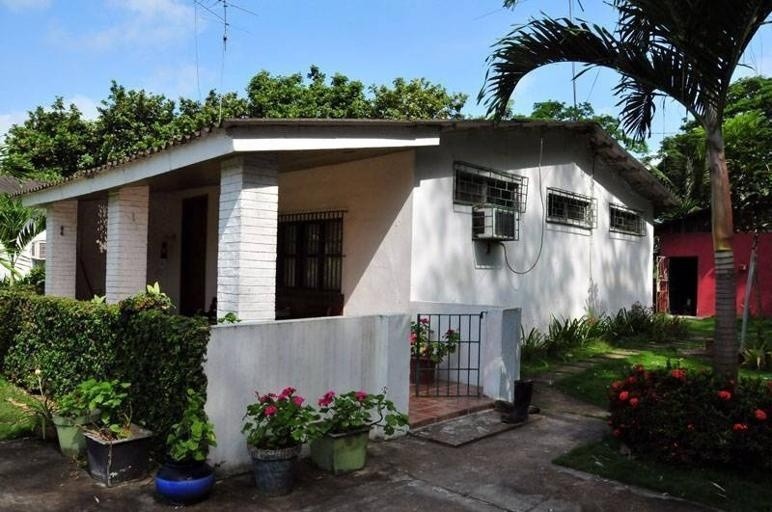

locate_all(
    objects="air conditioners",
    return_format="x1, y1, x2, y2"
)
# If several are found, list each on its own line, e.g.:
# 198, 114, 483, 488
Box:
471, 206, 520, 255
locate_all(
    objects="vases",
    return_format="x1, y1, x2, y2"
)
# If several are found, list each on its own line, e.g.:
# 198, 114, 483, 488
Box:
245, 420, 375, 497
409, 355, 436, 385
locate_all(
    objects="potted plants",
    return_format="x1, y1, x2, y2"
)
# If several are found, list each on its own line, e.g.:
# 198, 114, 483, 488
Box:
51, 375, 221, 508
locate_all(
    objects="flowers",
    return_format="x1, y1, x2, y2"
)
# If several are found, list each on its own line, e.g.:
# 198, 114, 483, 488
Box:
234, 384, 416, 443
409, 312, 463, 366
607, 361, 770, 456
